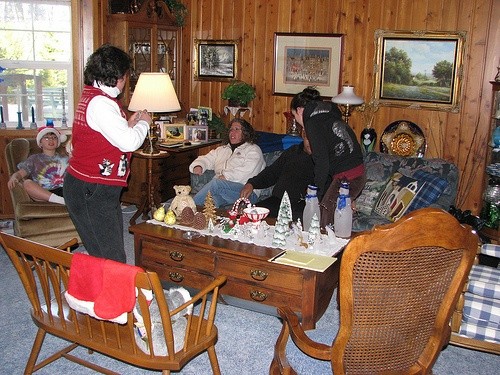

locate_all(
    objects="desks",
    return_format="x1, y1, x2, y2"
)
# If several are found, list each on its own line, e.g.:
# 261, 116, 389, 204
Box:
121, 138, 223, 206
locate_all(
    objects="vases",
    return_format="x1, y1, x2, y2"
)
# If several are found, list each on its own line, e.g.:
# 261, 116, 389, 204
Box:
361, 128, 378, 156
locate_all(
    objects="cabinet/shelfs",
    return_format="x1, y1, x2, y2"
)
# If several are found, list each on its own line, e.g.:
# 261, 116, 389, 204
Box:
105, 0, 190, 120
479, 66, 500, 246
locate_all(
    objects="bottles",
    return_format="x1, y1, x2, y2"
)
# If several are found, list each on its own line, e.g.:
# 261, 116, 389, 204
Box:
481, 178, 500, 229
334, 178, 352, 238
303, 184, 321, 232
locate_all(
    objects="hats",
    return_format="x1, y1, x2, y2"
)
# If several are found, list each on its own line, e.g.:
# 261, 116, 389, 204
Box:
36, 126, 67, 149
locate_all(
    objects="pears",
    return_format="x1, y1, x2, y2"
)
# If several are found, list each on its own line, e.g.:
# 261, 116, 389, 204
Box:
153, 206, 176, 225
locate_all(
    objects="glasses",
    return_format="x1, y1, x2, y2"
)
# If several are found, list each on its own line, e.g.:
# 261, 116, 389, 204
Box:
230, 127, 243, 132
43, 136, 58, 141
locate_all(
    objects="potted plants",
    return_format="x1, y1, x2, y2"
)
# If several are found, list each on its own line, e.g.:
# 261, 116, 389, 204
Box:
221, 81, 256, 107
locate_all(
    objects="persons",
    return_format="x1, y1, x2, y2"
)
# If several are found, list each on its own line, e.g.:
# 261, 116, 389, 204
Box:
290, 87, 366, 234
188, 118, 266, 207
241, 129, 333, 224
63, 45, 152, 263
7, 126, 70, 204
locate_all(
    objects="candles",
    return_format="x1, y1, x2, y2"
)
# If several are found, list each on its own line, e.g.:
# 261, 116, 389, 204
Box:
0, 88, 65, 123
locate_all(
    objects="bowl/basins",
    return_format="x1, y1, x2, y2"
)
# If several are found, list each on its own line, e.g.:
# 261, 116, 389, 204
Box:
243, 207, 270, 220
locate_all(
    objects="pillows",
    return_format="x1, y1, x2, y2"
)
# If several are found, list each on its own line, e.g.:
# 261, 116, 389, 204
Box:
355, 171, 449, 222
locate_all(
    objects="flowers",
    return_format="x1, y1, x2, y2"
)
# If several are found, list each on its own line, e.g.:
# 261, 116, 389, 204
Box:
356, 97, 379, 129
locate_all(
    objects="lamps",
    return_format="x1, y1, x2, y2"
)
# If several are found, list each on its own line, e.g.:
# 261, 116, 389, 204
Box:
330, 86, 364, 124
128, 72, 181, 225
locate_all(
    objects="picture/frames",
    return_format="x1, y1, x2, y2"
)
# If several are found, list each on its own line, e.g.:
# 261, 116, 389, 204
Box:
369, 29, 468, 113
271, 32, 345, 100
155, 107, 213, 143
193, 39, 241, 82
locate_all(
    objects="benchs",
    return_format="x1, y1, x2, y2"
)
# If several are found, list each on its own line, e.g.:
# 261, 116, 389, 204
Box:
0, 230, 227, 375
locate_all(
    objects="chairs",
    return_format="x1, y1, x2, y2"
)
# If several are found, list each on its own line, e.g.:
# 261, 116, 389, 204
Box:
449, 244, 500, 355
270, 207, 472, 375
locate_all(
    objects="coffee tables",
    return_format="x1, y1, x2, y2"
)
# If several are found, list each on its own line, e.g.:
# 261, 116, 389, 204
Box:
129, 205, 357, 331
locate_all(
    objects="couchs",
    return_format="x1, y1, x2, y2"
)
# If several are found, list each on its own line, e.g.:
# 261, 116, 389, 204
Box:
190, 131, 459, 232
5, 138, 83, 247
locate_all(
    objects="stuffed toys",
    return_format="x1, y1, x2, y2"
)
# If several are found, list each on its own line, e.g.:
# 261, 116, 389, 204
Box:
134, 288, 193, 356
169, 185, 196, 221
